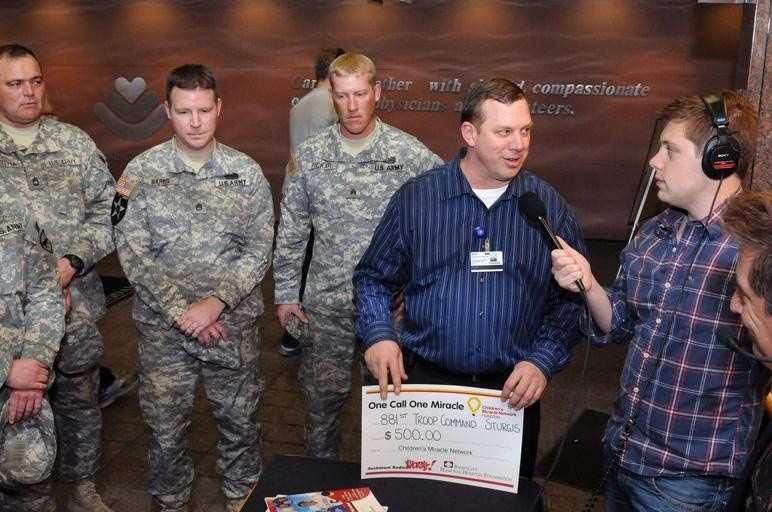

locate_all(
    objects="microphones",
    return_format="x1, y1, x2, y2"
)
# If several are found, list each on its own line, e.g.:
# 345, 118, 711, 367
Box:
716, 326, 772, 363
519, 192, 585, 292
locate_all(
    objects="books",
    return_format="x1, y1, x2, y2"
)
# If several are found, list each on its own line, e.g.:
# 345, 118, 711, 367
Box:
263, 487, 389, 512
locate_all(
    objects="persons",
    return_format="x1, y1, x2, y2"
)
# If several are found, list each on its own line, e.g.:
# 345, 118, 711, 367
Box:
273, 52, 445, 460
352, 78, 589, 482
111, 63, 275, 512
0, 175, 67, 511
719, 192, 771, 510
551, 89, 765, 512
277, 47, 345, 358
0, 45, 118, 511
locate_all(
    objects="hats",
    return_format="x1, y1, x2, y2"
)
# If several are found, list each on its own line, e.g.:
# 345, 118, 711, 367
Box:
0, 398, 57, 487
182, 322, 243, 370
284, 313, 316, 347
58, 313, 105, 375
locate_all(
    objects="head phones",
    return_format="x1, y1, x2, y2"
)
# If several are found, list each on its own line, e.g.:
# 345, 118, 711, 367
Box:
698, 92, 740, 180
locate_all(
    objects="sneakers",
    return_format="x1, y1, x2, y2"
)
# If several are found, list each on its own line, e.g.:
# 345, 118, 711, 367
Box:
278, 332, 301, 356
100, 371, 139, 408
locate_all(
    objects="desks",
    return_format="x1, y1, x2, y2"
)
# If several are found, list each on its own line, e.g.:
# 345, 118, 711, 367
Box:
238, 452, 546, 511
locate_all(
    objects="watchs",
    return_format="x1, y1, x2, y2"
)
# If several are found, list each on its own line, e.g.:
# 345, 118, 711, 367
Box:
63, 254, 84, 272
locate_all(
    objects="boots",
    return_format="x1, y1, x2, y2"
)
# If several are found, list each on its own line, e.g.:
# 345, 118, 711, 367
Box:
63, 478, 112, 511
